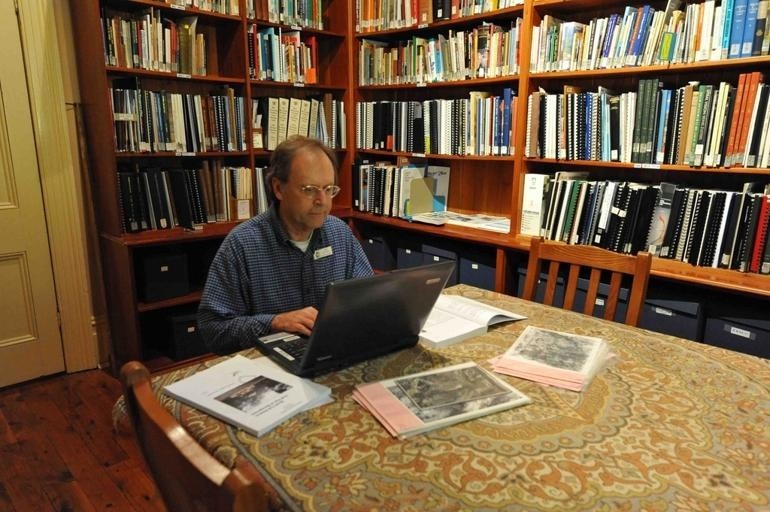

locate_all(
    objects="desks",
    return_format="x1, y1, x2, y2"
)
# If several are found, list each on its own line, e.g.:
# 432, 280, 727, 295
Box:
114, 285, 769, 511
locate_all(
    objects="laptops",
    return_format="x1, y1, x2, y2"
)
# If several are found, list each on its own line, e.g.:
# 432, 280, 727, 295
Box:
254, 259, 457, 378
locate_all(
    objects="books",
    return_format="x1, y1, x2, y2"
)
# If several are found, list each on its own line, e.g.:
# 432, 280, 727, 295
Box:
352, 361, 535, 444
495, 323, 608, 392
416, 292, 528, 347
163, 352, 332, 438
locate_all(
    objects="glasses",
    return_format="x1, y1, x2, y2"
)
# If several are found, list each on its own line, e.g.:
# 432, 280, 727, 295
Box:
285, 181, 341, 199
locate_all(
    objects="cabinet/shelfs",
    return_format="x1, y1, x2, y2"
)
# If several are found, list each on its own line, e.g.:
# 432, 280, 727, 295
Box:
61, 0, 769, 382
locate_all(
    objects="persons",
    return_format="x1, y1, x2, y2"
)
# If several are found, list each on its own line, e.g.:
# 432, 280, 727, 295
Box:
192, 135, 373, 351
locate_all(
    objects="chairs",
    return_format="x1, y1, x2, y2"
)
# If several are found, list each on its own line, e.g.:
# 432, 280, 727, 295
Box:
121, 356, 269, 511
